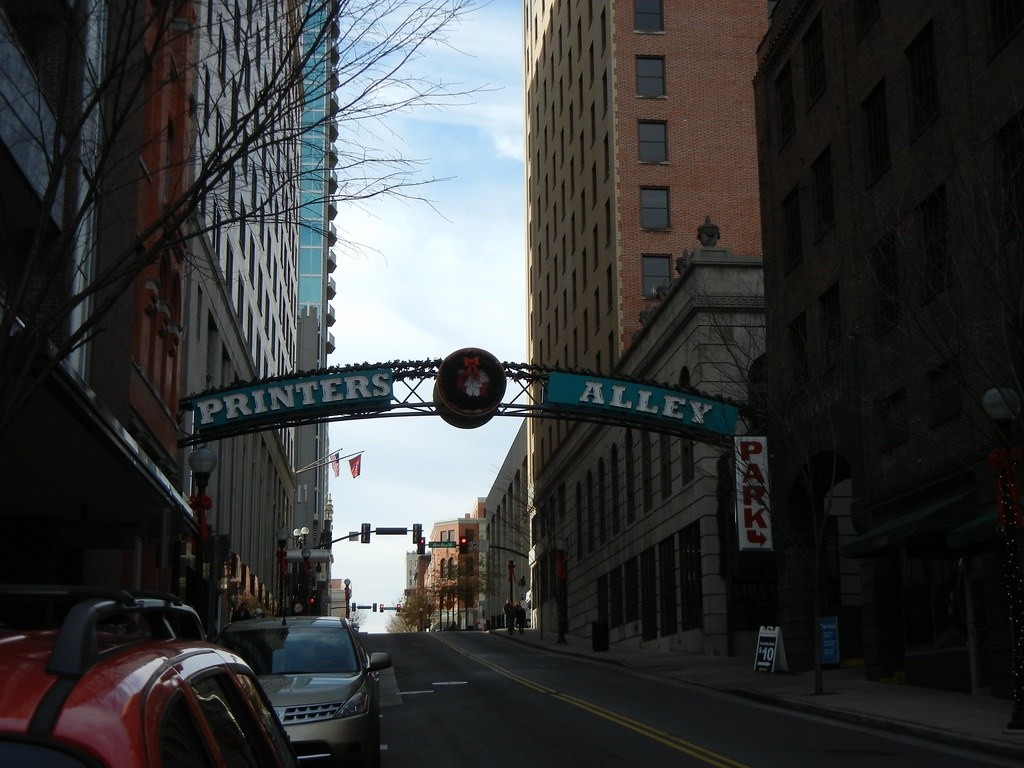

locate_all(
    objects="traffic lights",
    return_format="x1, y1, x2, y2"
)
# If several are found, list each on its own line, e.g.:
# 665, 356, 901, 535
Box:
397, 604, 400, 612
460, 536, 468, 555
381, 605, 384, 612
418, 537, 425, 553
309, 599, 316, 608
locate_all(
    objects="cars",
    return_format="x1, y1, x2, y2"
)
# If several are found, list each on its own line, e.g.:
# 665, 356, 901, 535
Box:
431, 621, 456, 632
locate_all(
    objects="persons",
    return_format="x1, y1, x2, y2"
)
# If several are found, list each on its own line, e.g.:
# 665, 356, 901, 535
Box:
505, 600, 522, 627
230, 603, 265, 622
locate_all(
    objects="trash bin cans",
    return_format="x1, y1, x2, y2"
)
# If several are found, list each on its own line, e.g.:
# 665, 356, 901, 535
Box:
591, 619, 609, 651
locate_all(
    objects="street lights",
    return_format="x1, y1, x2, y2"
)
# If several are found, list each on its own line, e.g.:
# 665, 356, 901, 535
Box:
294, 526, 310, 613
507, 553, 515, 602
983, 386, 1024, 729
277, 532, 288, 614
556, 539, 566, 645
345, 579, 351, 618
189, 445, 217, 630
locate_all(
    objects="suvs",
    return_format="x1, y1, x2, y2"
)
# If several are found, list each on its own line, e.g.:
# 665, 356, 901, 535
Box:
211, 616, 393, 768
0, 587, 333, 768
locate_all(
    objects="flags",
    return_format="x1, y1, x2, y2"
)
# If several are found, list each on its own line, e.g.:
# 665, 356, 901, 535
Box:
349, 454, 361, 479
330, 453, 340, 477
